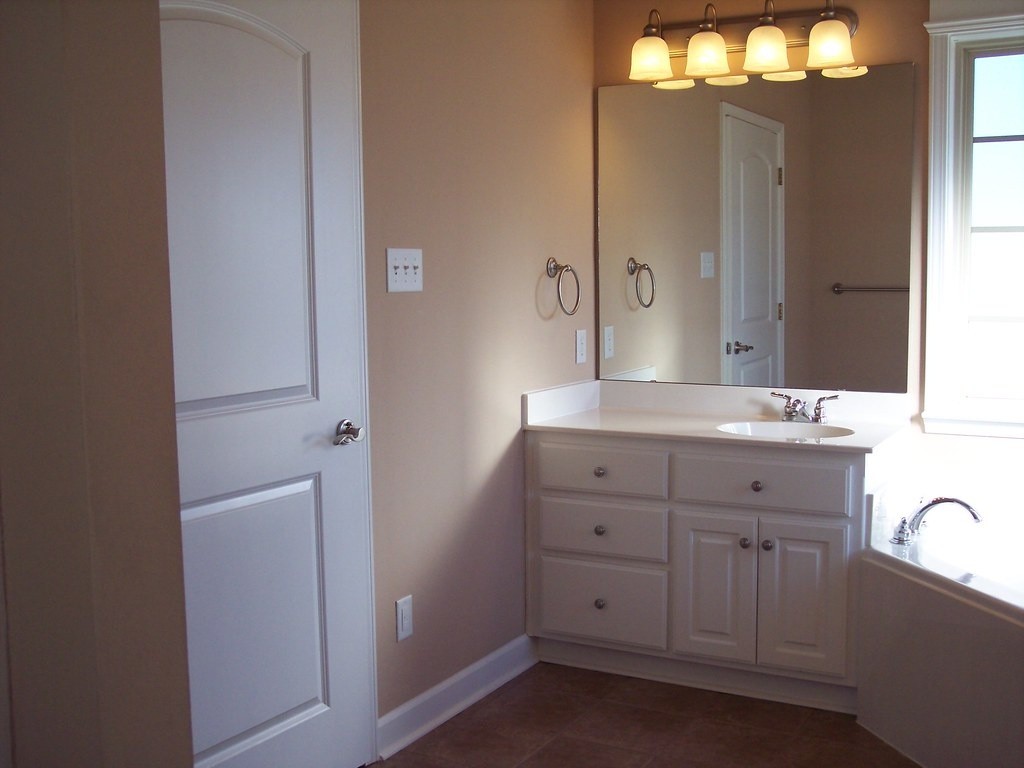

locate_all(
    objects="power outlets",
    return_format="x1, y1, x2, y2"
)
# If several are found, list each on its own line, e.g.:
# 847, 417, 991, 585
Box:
395, 593, 413, 643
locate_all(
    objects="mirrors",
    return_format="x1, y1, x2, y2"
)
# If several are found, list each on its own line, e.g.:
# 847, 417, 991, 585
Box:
597, 61, 916, 393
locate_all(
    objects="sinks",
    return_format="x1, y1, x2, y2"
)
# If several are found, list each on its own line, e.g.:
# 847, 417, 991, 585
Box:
715, 422, 855, 438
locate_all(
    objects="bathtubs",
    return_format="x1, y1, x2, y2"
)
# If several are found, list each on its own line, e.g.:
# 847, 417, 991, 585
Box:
853, 494, 1022, 766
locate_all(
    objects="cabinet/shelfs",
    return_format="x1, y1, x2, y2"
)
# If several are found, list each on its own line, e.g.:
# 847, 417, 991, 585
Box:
525, 430, 913, 716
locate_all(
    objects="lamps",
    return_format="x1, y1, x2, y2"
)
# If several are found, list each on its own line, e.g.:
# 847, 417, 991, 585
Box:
743, 0, 789, 72
629, 9, 674, 81
685, 4, 731, 77
806, 0, 855, 67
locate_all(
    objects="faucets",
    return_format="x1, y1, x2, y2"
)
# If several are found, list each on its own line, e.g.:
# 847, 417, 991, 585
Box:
790, 399, 812, 422
910, 497, 983, 535
